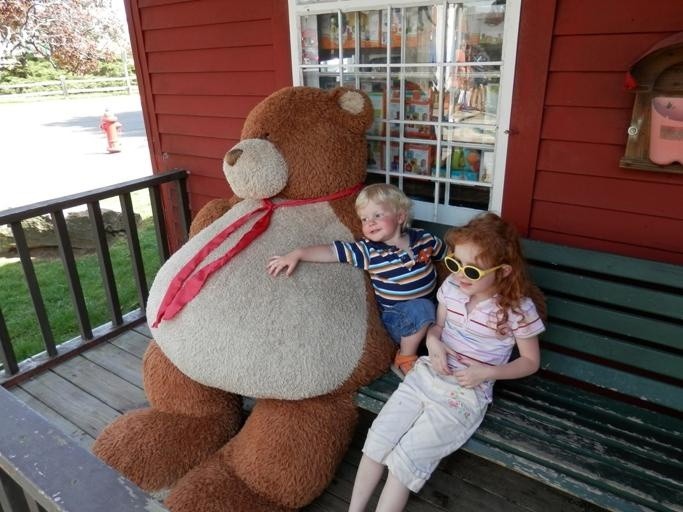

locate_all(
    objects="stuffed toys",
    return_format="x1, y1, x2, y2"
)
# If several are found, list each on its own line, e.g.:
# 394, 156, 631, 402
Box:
91, 86, 396, 512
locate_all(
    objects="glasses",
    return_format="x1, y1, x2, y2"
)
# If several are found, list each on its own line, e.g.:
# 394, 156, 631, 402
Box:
443, 253, 503, 281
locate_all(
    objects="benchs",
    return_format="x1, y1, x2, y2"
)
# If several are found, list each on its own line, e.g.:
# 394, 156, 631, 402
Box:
357, 220, 683, 512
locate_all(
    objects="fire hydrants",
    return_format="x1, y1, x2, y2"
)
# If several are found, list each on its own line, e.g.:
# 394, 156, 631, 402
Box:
99, 108, 123, 154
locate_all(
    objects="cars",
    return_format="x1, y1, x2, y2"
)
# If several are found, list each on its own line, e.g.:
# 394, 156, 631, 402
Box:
301, 30, 437, 88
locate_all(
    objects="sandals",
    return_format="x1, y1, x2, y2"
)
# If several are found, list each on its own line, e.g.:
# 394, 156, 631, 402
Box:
390, 351, 419, 380
